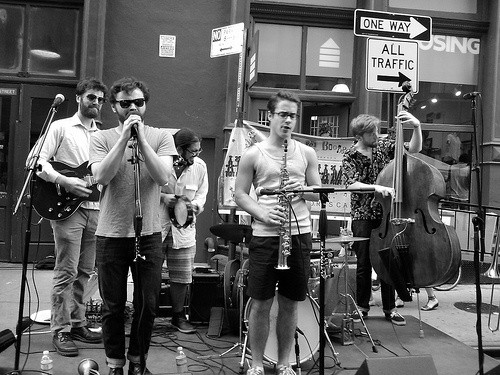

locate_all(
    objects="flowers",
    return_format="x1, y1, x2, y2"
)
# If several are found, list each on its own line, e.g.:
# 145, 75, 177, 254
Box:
318, 122, 333, 133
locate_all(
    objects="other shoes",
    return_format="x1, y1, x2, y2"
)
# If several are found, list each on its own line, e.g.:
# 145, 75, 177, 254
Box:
127, 360, 153, 375
246, 367, 264, 375
109, 368, 124, 375
351, 310, 368, 322
395, 297, 404, 307
170, 315, 197, 334
125, 322, 132, 335
385, 311, 407, 325
275, 362, 296, 375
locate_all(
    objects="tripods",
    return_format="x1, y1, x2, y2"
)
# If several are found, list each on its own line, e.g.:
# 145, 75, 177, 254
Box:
325, 242, 376, 352
217, 241, 253, 358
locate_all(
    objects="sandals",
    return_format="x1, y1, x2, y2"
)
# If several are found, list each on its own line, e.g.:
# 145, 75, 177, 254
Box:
421, 296, 440, 311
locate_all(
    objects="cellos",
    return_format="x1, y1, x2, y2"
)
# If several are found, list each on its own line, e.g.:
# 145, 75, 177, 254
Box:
368, 80, 462, 338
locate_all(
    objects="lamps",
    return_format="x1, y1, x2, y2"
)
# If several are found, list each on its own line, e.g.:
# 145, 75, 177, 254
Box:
332, 79, 349, 92
452, 89, 461, 96
30, 35, 60, 58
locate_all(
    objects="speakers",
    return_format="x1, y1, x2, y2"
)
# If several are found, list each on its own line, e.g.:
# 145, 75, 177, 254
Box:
355, 353, 438, 375
188, 272, 223, 324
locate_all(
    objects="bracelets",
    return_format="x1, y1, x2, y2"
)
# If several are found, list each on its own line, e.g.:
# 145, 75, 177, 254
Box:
413, 124, 419, 128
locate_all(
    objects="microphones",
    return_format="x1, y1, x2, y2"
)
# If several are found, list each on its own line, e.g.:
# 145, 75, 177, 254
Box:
462, 91, 480, 99
125, 112, 144, 129
52, 94, 65, 108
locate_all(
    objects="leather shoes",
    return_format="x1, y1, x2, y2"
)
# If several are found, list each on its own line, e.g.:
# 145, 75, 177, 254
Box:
51, 332, 79, 357
70, 326, 103, 343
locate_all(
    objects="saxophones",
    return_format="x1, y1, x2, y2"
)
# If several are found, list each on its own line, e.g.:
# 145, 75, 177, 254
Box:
274, 139, 291, 271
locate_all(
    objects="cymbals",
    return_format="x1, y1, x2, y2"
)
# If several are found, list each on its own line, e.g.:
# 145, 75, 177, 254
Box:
325, 235, 371, 244
209, 223, 253, 245
168, 194, 193, 229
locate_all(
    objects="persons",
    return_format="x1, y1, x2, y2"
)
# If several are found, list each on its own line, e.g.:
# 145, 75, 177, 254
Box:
88, 77, 180, 375
26, 79, 103, 357
445, 132, 461, 193
342, 111, 440, 326
234, 90, 321, 375
158, 126, 210, 333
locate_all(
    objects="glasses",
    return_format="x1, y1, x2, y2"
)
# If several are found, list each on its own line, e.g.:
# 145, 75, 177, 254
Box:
181, 147, 203, 156
86, 94, 106, 105
115, 98, 146, 109
271, 112, 299, 120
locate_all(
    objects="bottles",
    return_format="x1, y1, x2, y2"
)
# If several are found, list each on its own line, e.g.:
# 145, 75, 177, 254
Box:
176, 346, 188, 373
40, 350, 54, 375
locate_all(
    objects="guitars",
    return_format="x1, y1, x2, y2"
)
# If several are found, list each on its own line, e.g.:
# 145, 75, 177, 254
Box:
28, 155, 187, 222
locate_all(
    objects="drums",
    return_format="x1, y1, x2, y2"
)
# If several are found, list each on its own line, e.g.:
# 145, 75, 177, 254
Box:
308, 258, 335, 284
244, 284, 327, 365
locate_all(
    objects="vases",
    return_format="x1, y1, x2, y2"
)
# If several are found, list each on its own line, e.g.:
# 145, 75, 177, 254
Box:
321, 133, 329, 136
